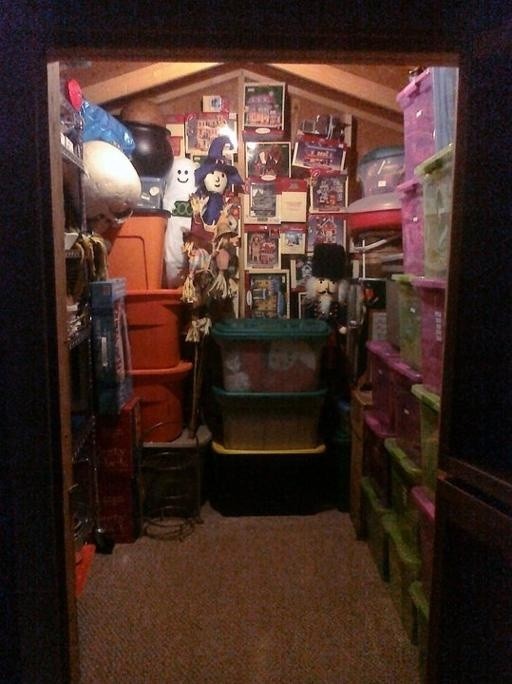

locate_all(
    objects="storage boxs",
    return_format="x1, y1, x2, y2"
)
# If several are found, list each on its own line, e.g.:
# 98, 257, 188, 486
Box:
186, 76, 348, 516
348, 144, 407, 581
383, 59, 459, 680
47, 63, 203, 552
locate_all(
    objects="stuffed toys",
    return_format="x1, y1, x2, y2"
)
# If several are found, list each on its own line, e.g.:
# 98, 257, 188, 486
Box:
163, 135, 246, 341
302, 242, 348, 370
